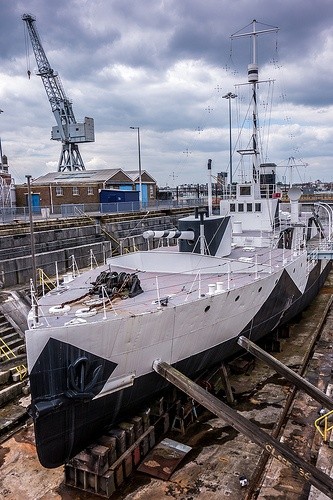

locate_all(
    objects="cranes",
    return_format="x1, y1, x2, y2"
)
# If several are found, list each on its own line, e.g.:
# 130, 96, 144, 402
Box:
21, 12, 95, 172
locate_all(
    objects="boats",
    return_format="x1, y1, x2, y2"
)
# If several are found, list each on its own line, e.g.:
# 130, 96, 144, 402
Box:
26, 161, 333, 470
